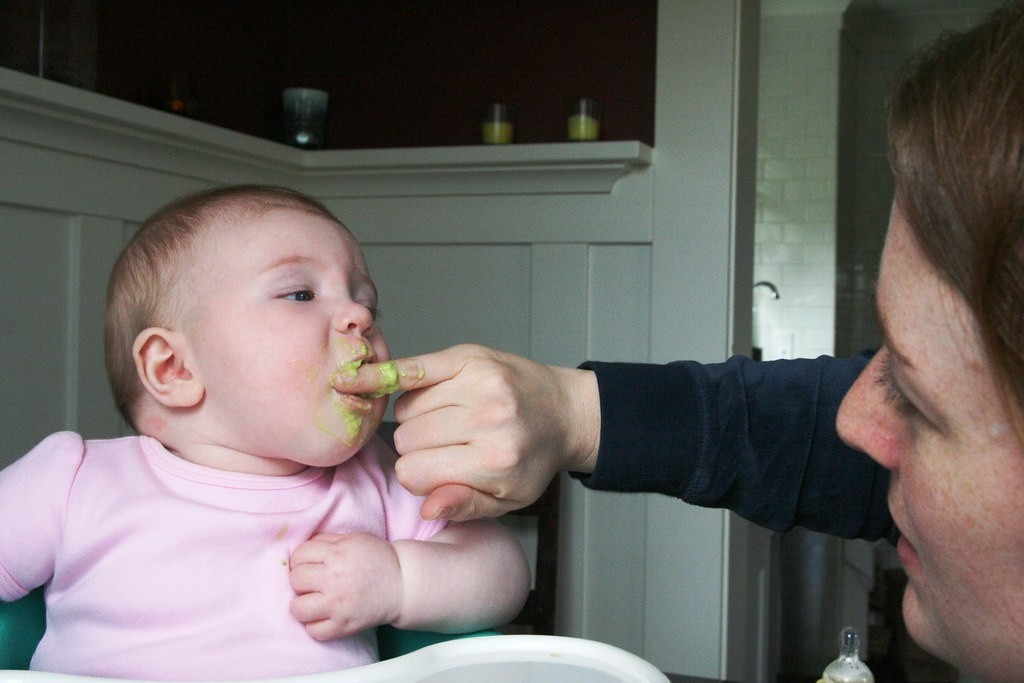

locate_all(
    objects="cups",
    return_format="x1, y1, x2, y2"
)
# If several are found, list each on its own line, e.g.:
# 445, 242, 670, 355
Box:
566, 98, 604, 141
480, 102, 516, 145
279, 87, 332, 151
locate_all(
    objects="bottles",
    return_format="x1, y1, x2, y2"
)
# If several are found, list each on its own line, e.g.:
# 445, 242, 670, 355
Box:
816, 627, 875, 683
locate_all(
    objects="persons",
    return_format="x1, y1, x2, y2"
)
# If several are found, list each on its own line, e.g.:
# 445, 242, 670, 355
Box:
0, 180, 534, 683
327, 0, 1024, 683
